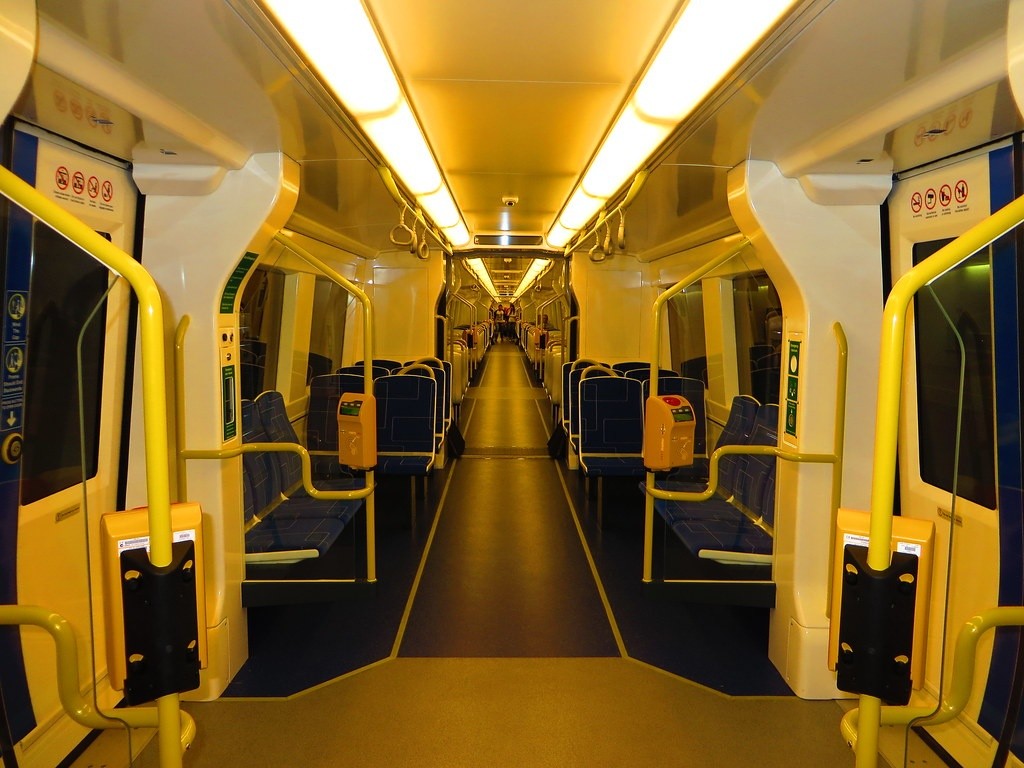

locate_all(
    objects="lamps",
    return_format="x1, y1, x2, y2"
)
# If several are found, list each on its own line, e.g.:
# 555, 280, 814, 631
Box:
254, 0, 802, 306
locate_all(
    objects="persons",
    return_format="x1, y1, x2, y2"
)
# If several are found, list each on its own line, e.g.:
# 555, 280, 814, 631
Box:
539, 314, 553, 329
508, 304, 516, 340
493, 305, 507, 343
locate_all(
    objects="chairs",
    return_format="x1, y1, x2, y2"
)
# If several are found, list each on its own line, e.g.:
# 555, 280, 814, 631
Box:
242, 312, 497, 600
514, 318, 816, 595
